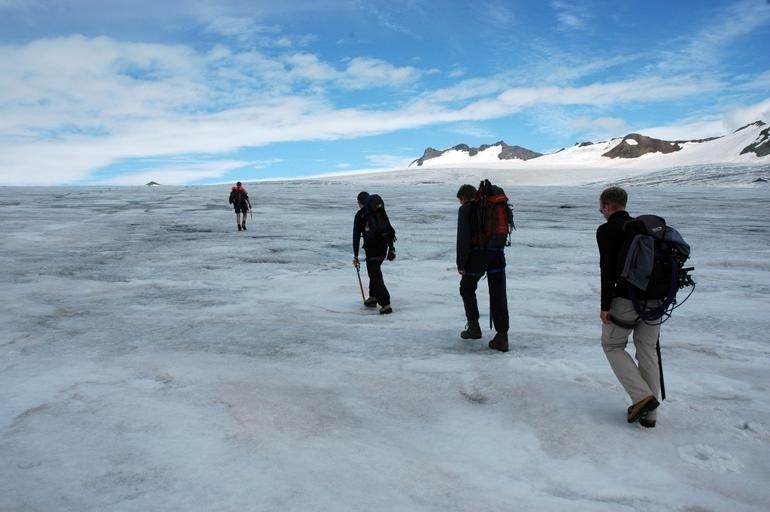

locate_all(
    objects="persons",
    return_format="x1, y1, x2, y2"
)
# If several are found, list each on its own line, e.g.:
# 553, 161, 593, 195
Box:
456, 183, 510, 352
596, 185, 669, 429
352, 190, 397, 315
229, 181, 253, 232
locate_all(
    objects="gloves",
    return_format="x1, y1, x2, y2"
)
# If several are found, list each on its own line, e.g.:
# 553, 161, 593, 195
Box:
387, 246, 396, 261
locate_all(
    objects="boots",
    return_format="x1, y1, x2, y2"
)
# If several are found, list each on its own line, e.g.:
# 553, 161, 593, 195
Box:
489, 334, 508, 352
461, 321, 481, 339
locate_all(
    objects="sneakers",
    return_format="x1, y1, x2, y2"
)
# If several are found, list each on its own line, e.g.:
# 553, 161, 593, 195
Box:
638, 409, 657, 427
242, 223, 246, 230
380, 304, 392, 314
628, 395, 659, 423
365, 296, 377, 307
238, 225, 242, 231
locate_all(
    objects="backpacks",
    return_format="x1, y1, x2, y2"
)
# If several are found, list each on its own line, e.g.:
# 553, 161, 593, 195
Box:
611, 215, 690, 291
471, 179, 516, 247
365, 194, 397, 243
231, 187, 244, 204
